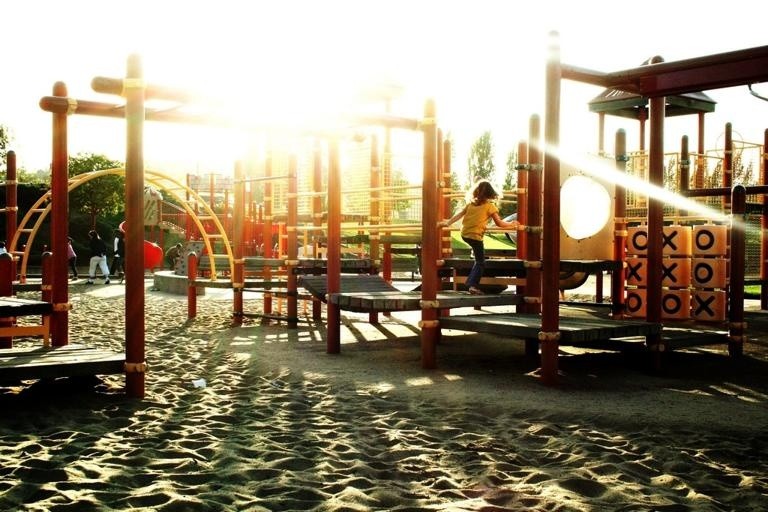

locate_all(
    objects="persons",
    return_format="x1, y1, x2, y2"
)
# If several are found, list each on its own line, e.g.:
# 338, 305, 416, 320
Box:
436, 181, 521, 295
68, 236, 78, 280
86, 230, 111, 284
165, 243, 183, 271
109, 229, 125, 277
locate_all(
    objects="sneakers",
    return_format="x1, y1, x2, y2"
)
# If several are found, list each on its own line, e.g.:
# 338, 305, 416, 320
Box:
150, 287, 160, 291
105, 280, 109, 284
72, 277, 78, 280
86, 281, 94, 284
469, 287, 484, 295
